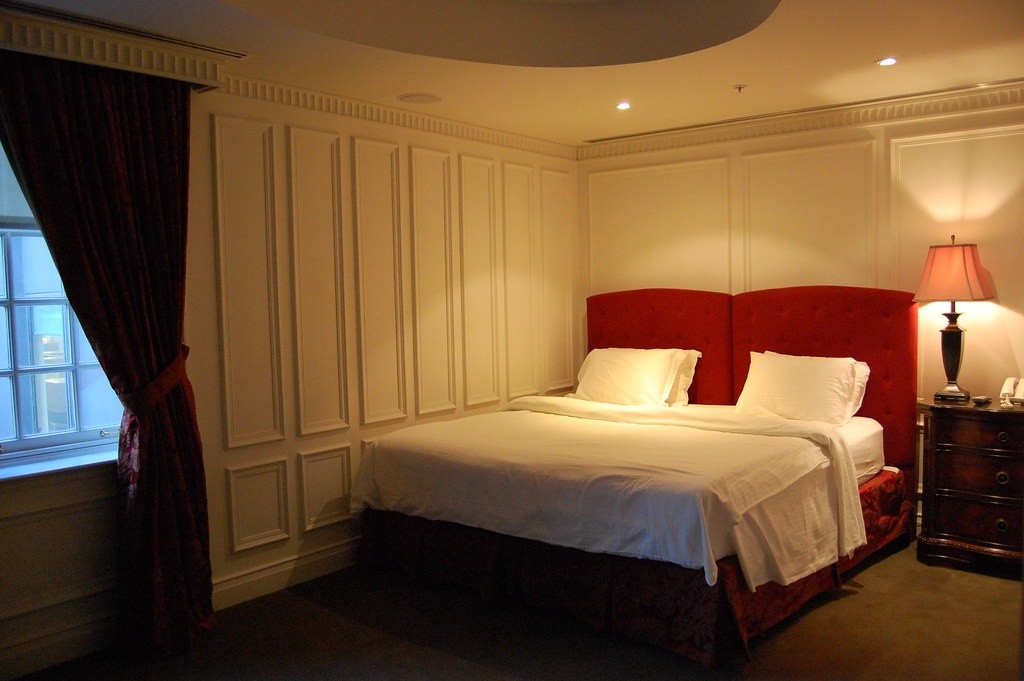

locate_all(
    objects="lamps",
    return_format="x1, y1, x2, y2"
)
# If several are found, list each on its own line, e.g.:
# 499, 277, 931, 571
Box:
912, 235, 994, 403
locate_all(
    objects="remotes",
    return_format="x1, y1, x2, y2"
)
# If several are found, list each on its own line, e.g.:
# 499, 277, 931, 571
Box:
971, 396, 992, 404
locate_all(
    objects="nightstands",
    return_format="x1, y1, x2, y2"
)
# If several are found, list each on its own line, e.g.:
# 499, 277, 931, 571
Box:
916, 399, 1023, 582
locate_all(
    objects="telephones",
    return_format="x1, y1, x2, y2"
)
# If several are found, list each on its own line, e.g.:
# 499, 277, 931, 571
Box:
998, 376, 1024, 403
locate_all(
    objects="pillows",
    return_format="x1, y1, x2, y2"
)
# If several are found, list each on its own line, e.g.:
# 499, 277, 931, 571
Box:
350, 284, 918, 670
734, 352, 857, 428
667, 349, 702, 409
763, 350, 871, 426
563, 347, 686, 408
